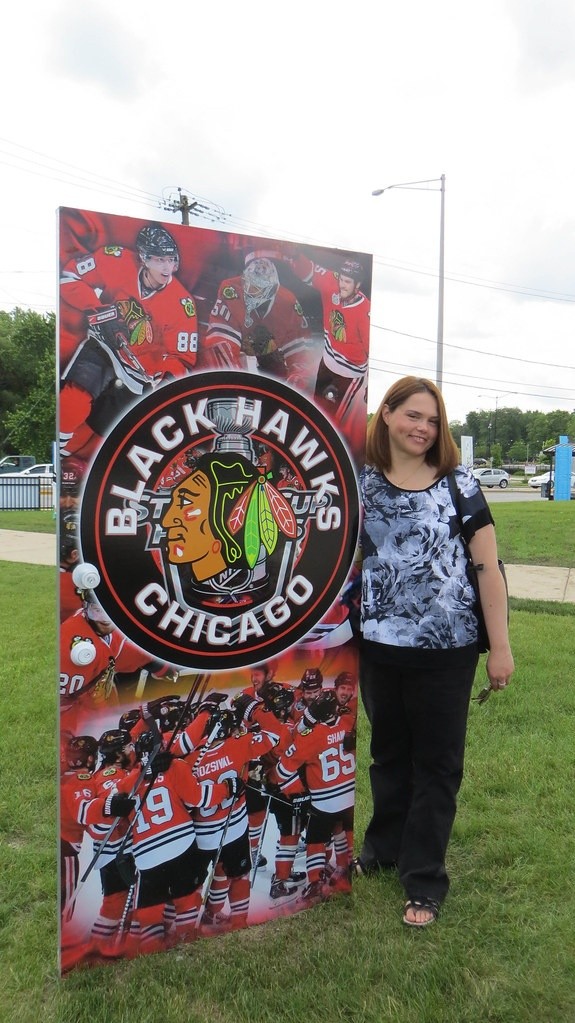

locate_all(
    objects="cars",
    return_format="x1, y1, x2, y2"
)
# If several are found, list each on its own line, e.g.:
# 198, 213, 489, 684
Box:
473, 467, 511, 488
527, 470, 555, 489
473, 457, 487, 465
19, 463, 54, 474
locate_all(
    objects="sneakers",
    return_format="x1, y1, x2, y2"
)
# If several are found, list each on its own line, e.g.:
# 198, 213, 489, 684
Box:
267, 873, 298, 910
323, 860, 336, 880
248, 848, 268, 871
295, 872, 328, 905
200, 909, 230, 931
292, 839, 309, 859
284, 871, 306, 888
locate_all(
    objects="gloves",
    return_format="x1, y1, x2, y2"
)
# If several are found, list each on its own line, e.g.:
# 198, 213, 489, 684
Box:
303, 698, 330, 728
140, 753, 172, 780
86, 304, 130, 350
223, 777, 245, 800
102, 793, 137, 818
139, 694, 180, 720
198, 692, 228, 715
230, 694, 260, 722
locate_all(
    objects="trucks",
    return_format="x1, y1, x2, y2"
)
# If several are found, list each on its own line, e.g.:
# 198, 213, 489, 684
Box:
0, 454, 36, 474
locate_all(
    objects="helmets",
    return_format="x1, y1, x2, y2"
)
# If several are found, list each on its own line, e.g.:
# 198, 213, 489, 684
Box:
60, 729, 75, 773
301, 669, 324, 689
97, 729, 132, 757
136, 225, 179, 271
59, 457, 116, 634
204, 709, 239, 740
65, 736, 98, 769
262, 683, 295, 716
242, 256, 280, 328
118, 709, 143, 732
334, 672, 358, 689
134, 728, 162, 762
338, 259, 366, 290
251, 655, 279, 680
159, 701, 193, 734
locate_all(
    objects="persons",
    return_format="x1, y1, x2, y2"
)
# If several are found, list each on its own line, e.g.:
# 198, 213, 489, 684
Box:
202, 257, 317, 393
61, 462, 179, 739
350, 375, 515, 926
61, 662, 360, 956
273, 242, 370, 415
57, 223, 198, 456
11, 459, 18, 466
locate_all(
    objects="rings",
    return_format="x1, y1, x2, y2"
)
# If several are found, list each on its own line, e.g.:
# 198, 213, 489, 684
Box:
499, 683, 506, 685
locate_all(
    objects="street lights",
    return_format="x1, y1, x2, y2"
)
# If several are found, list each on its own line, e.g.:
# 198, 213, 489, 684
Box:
371, 172, 447, 398
477, 391, 518, 457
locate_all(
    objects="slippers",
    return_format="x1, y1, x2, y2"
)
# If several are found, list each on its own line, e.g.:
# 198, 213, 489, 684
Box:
403, 897, 441, 926
352, 857, 367, 877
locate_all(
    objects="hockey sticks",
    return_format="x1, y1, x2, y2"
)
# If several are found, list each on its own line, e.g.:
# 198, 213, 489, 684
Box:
198, 671, 212, 704
115, 867, 137, 946
192, 720, 222, 774
116, 673, 202, 859
117, 333, 159, 390
249, 797, 273, 889
62, 741, 163, 919
193, 763, 247, 934
135, 670, 149, 701
